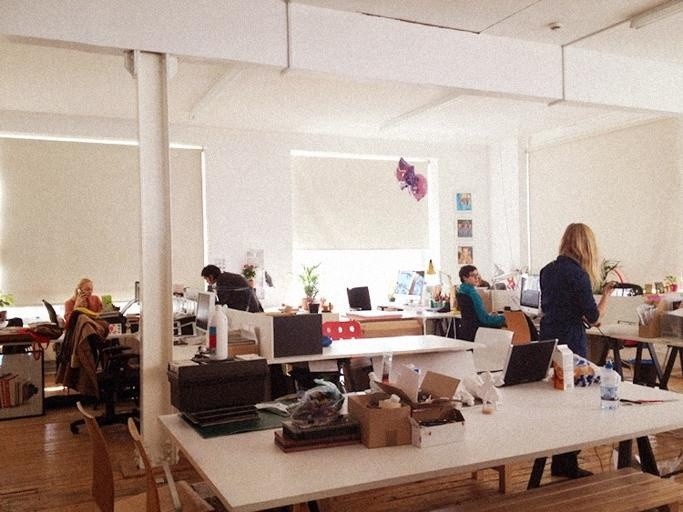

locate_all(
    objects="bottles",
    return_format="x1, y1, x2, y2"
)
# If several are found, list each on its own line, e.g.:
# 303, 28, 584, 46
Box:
599, 359, 621, 410
383, 393, 401, 409
367, 371, 383, 392
208, 304, 230, 361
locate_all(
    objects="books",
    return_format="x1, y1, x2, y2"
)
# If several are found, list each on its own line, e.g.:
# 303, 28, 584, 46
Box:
269, 417, 361, 452
227, 333, 256, 345
0, 372, 37, 408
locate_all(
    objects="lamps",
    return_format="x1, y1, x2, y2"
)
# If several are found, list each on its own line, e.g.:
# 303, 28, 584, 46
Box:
427, 259, 452, 288
629, 0, 683, 31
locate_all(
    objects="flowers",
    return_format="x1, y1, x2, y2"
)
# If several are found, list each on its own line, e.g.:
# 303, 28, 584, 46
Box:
240, 262, 257, 280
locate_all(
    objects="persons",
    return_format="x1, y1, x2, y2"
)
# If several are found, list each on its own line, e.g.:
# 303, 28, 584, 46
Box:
455, 265, 506, 339
200, 264, 264, 313
460, 247, 472, 264
457, 193, 469, 211
458, 220, 470, 237
537, 223, 616, 476
245, 276, 256, 293
458, 252, 469, 264
64, 278, 109, 337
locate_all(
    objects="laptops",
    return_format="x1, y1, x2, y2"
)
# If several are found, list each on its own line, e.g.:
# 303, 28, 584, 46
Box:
497, 338, 559, 387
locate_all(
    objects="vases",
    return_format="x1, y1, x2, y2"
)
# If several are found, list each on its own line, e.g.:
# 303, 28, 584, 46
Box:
245, 278, 254, 289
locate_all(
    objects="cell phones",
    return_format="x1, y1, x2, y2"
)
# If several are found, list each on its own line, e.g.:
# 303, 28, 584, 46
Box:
77, 288, 82, 295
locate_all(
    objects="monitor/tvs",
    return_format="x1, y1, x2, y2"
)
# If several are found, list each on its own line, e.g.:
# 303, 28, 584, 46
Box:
194, 291, 215, 333
518, 274, 542, 314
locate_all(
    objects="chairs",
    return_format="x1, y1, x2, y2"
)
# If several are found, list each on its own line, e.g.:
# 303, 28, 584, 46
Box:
1, 292, 142, 434
77, 402, 214, 511
156, 287, 682, 511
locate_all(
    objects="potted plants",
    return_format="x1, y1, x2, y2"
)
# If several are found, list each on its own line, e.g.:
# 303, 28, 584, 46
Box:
297, 260, 322, 310
664, 275, 678, 291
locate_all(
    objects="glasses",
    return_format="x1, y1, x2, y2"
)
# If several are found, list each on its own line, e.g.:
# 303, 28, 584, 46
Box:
469, 273, 480, 277
